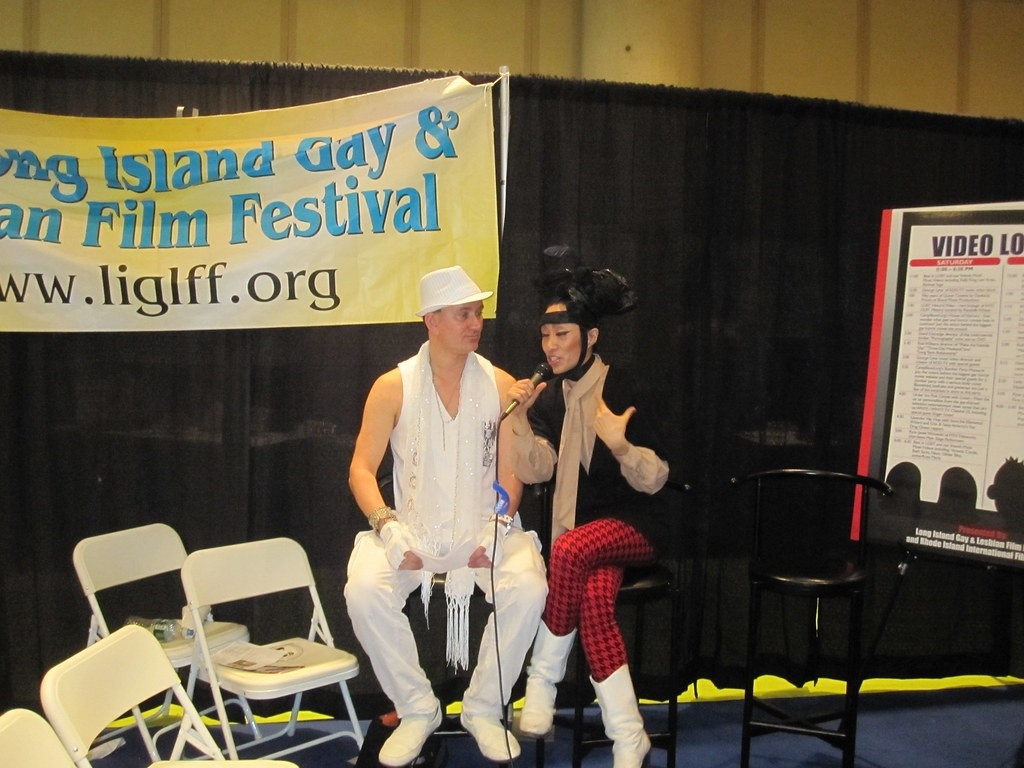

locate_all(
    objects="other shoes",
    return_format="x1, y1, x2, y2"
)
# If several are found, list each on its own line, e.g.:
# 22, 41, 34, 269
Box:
378, 697, 443, 767
460, 708, 522, 762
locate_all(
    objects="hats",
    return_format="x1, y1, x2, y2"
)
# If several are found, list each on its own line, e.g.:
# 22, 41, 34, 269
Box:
415, 265, 493, 318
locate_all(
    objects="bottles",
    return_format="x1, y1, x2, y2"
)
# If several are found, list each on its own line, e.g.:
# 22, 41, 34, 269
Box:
125, 615, 196, 643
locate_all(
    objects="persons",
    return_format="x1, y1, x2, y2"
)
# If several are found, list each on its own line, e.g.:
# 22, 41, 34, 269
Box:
508, 247, 675, 768
344, 264, 549, 768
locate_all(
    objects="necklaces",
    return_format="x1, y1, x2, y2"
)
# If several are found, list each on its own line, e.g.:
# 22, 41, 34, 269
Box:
430, 372, 459, 450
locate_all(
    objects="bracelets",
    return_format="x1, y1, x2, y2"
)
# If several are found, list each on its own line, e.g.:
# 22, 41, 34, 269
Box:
487, 514, 513, 526
368, 505, 392, 529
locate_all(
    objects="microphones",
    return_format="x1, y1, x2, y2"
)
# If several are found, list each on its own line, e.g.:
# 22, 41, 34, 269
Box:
500, 363, 552, 421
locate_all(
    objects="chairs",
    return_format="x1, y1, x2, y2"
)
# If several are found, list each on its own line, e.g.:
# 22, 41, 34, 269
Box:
72, 523, 262, 761
168, 537, 363, 768
531, 475, 696, 768
361, 473, 514, 768
0, 624, 299, 768
720, 470, 893, 768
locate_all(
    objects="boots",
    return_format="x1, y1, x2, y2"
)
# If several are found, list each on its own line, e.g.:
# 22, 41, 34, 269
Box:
521, 618, 577, 739
588, 664, 651, 768
